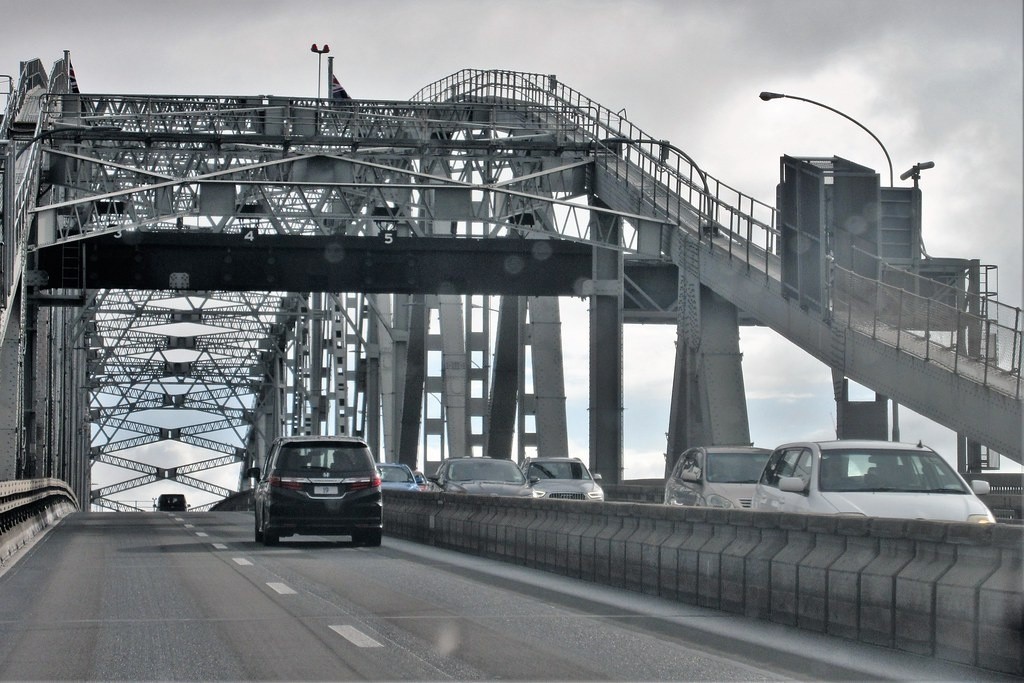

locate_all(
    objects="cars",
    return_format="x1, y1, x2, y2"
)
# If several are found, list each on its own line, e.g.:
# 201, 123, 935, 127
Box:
520, 457, 605, 502
246, 434, 382, 546
752, 439, 997, 524
664, 446, 774, 509
426, 457, 540, 497
376, 462, 421, 492
413, 471, 429, 492
153, 493, 191, 512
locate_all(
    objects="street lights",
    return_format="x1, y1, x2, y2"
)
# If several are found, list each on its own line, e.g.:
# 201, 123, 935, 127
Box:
761, 90, 900, 442
151, 497, 157, 512
